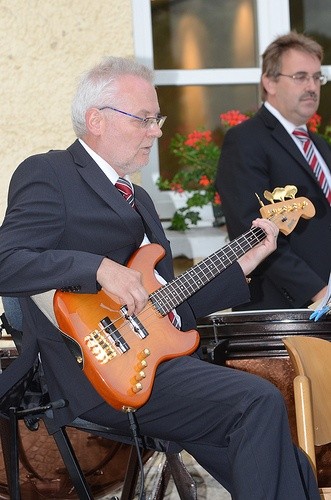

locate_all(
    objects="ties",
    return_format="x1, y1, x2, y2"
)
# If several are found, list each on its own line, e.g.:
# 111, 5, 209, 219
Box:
293, 127, 331, 208
115, 177, 182, 333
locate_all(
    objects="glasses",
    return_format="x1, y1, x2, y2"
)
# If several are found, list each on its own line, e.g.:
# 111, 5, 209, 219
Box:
100, 106, 167, 129
267, 72, 328, 87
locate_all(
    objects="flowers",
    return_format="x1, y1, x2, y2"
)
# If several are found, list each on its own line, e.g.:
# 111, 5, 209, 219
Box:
157, 109, 331, 230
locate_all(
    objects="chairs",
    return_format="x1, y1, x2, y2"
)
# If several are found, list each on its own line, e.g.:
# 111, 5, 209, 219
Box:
281, 334, 331, 500
0, 296, 197, 500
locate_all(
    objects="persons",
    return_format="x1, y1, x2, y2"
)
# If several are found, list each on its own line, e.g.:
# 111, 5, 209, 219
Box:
207, 27, 331, 312
0, 57, 324, 500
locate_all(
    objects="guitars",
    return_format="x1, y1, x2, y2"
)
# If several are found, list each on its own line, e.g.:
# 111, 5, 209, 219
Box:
49, 184, 316, 414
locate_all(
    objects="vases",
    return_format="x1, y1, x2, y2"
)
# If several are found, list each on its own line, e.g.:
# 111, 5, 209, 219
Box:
212, 202, 226, 227
